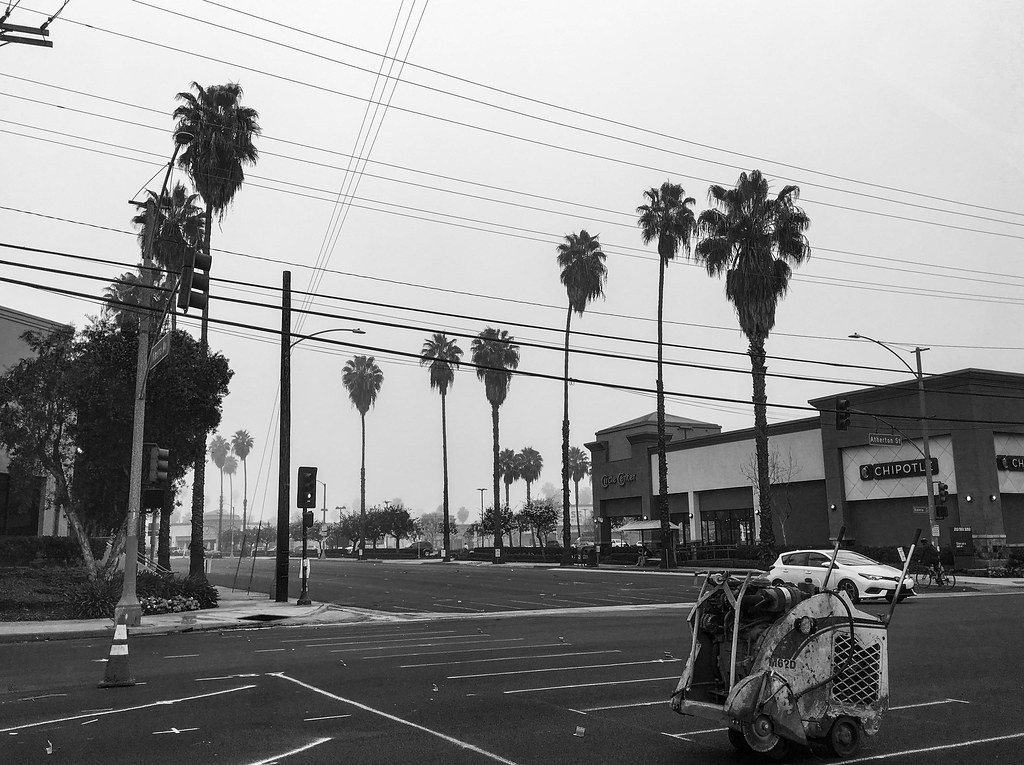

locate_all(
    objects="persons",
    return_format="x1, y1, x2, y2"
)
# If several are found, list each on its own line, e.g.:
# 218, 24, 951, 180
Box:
920, 536, 944, 584
463, 543, 468, 552
636, 546, 650, 567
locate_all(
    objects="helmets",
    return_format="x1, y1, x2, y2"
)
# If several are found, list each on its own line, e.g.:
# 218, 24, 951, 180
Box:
921, 538, 927, 543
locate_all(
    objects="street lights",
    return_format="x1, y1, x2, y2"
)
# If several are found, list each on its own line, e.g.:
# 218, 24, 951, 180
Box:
848, 332, 944, 581
274, 270, 366, 602
335, 506, 346, 529
477, 488, 488, 547
115, 133, 194, 625
581, 509, 588, 531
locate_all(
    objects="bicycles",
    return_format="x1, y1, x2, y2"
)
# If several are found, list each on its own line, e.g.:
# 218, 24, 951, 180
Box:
915, 560, 956, 590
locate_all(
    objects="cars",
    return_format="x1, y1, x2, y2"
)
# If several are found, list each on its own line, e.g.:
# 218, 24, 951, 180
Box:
204, 551, 222, 559
610, 539, 630, 548
767, 550, 917, 604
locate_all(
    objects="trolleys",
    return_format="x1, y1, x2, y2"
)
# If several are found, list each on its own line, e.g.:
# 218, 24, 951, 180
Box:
671, 526, 922, 759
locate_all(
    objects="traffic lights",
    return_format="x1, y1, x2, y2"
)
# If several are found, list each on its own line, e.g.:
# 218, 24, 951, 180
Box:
835, 395, 851, 431
936, 506, 948, 517
938, 483, 949, 505
149, 448, 169, 485
296, 466, 317, 508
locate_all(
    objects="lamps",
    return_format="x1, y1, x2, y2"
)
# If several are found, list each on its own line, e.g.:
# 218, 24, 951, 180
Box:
689, 513, 693, 518
755, 509, 759, 515
991, 495, 996, 502
830, 504, 836, 511
966, 495, 972, 502
643, 516, 648, 520
594, 519, 597, 523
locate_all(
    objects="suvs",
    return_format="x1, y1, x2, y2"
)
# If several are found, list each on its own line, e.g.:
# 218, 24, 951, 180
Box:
408, 541, 434, 557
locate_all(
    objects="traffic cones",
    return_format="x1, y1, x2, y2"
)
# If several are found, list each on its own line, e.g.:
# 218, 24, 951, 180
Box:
97, 614, 136, 688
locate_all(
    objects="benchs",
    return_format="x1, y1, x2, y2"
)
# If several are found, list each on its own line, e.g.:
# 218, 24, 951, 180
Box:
640, 549, 663, 566
